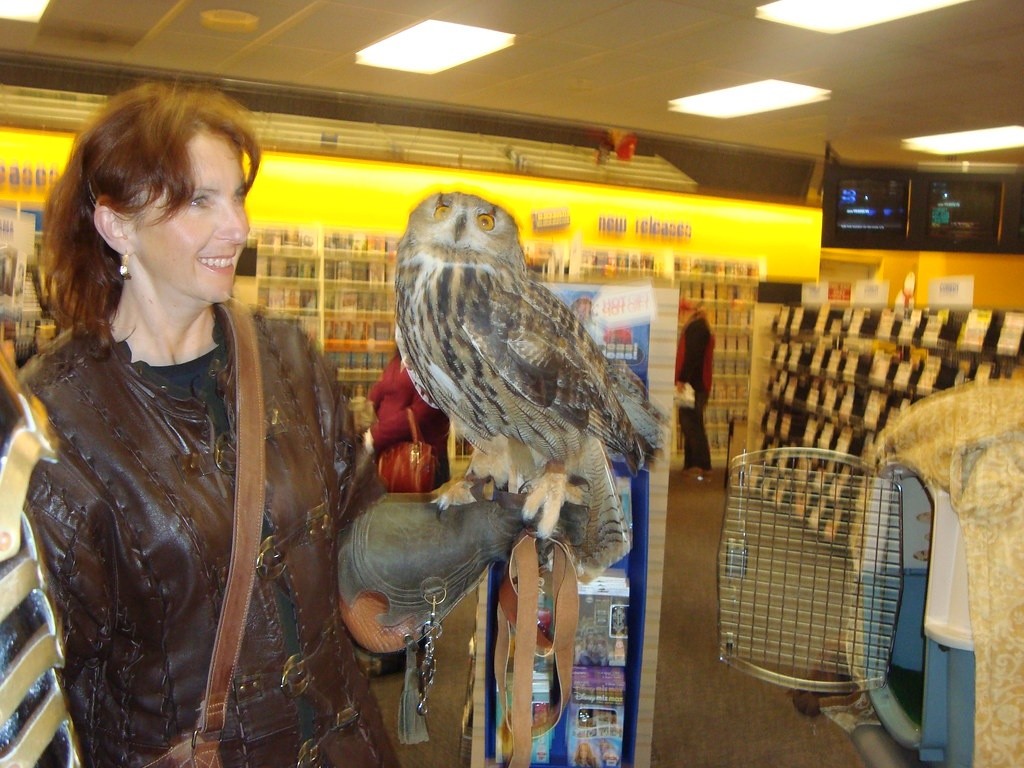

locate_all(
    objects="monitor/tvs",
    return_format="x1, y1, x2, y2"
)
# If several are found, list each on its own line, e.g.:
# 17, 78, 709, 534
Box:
821, 164, 1005, 253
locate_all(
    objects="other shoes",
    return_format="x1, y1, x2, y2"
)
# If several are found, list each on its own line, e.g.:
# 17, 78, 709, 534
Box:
680, 468, 714, 483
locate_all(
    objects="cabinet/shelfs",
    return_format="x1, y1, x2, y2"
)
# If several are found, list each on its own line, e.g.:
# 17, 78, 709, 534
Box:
470, 280, 682, 768
247, 226, 758, 470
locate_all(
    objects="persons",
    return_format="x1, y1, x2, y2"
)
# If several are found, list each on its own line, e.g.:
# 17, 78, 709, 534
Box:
674, 297, 715, 484
17, 81, 588, 768
362, 349, 451, 676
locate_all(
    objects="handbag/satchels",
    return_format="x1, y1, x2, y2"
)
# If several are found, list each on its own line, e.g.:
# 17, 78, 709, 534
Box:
379, 407, 438, 493
124, 730, 223, 768
